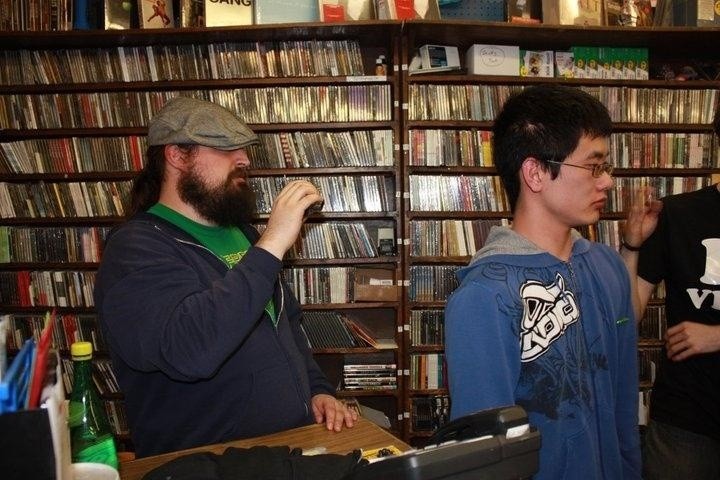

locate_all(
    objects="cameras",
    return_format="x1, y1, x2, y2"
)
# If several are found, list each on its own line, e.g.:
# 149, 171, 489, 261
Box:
304, 200, 325, 219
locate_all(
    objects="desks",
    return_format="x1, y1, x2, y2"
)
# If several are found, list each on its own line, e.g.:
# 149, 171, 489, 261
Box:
118, 412, 414, 480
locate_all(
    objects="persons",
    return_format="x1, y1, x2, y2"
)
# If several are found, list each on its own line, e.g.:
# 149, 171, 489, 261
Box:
144, 0, 170, 28
620, 103, 718, 480
442, 82, 646, 479
92, 95, 360, 463
564, 57, 574, 71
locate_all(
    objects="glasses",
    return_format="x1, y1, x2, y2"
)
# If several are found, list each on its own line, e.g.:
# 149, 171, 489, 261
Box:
544, 157, 616, 178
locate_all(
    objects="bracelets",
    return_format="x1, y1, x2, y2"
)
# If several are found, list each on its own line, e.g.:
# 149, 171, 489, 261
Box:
618, 235, 643, 253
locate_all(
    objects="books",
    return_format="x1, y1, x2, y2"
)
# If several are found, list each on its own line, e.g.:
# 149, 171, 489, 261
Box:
1, 0, 719, 429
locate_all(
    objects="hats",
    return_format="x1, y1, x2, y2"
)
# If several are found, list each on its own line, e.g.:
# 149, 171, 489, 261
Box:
146, 95, 262, 152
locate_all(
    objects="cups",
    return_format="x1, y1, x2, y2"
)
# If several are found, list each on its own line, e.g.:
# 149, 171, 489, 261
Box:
66, 462, 118, 479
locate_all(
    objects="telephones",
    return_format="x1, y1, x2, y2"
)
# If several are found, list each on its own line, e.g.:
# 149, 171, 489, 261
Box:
358, 402, 543, 480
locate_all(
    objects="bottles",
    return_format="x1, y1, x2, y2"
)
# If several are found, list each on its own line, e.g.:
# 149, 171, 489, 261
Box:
68, 342, 119, 472
375, 55, 387, 76
